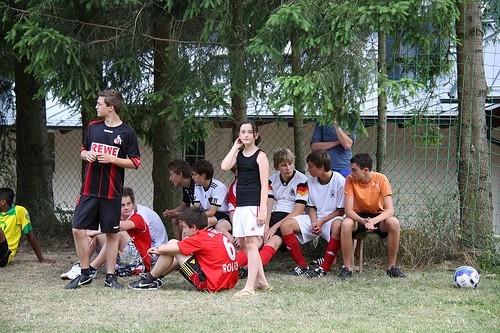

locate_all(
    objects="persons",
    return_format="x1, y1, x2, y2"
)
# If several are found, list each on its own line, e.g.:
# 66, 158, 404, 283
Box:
0, 187, 56, 268
161, 159, 198, 240
58, 188, 153, 281
178, 160, 231, 240
221, 119, 272, 298
127, 206, 239, 291
338, 153, 408, 279
215, 177, 274, 250
234, 147, 309, 279
281, 149, 350, 278
63, 90, 140, 288
133, 203, 171, 264
310, 112, 358, 179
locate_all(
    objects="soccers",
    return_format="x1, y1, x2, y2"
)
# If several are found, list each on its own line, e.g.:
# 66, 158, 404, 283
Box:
454, 265, 479, 289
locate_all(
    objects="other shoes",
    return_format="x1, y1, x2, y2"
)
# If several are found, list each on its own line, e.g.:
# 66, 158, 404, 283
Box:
238, 266, 247, 279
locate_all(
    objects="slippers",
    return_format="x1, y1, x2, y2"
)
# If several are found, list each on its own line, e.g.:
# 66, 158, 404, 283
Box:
254, 284, 273, 291
232, 290, 256, 297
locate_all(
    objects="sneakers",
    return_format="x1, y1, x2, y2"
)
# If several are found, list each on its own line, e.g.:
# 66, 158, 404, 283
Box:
60, 263, 97, 280
139, 272, 162, 288
311, 258, 324, 266
305, 267, 327, 278
64, 273, 92, 289
104, 274, 117, 288
128, 278, 158, 291
288, 264, 308, 276
337, 267, 352, 278
387, 266, 406, 278
